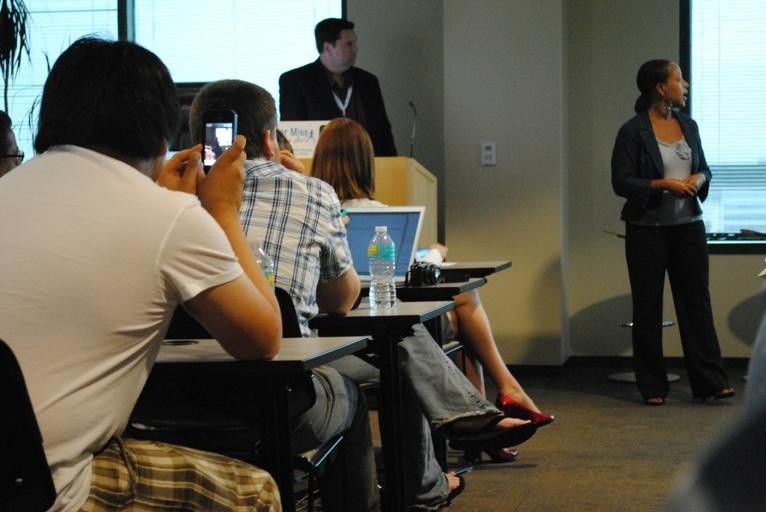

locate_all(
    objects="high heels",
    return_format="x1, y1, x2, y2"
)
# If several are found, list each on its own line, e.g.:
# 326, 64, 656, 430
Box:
494, 392, 556, 427
465, 447, 518, 465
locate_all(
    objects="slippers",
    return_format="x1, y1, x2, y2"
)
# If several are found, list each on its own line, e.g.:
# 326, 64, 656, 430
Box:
447, 414, 538, 452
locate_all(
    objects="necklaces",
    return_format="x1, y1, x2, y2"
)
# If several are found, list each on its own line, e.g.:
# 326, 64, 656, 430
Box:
652, 102, 671, 121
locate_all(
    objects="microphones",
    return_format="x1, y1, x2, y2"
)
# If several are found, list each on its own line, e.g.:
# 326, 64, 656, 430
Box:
407, 100, 418, 157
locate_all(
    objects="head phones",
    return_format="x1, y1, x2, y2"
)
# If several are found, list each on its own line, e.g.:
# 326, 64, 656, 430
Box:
411, 260, 441, 286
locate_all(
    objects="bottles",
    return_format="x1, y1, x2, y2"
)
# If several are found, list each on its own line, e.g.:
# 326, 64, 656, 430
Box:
367, 225, 397, 311
248, 238, 276, 292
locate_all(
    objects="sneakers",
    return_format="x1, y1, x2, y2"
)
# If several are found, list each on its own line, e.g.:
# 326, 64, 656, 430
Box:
443, 473, 465, 502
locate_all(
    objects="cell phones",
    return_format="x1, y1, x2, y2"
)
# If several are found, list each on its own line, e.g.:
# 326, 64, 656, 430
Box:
201, 108, 238, 174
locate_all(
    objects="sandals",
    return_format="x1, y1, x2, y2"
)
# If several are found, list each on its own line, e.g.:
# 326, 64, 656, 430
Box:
645, 397, 666, 406
701, 385, 736, 400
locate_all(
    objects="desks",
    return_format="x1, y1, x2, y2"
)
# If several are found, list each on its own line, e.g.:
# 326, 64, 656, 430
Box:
600, 224, 764, 384
155, 255, 516, 511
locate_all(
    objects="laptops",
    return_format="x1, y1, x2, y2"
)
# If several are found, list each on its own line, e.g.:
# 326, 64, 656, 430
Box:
344, 206, 426, 282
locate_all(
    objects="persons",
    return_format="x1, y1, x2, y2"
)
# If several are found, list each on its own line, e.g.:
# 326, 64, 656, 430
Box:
309, 117, 555, 464
187, 79, 380, 509
0, 110, 23, 178
275, 127, 539, 511
661, 289, 766, 512
0, 37, 285, 511
277, 18, 396, 158
610, 59, 734, 406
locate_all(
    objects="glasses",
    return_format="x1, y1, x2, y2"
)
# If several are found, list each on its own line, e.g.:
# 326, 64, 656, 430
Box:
2, 150, 26, 163
